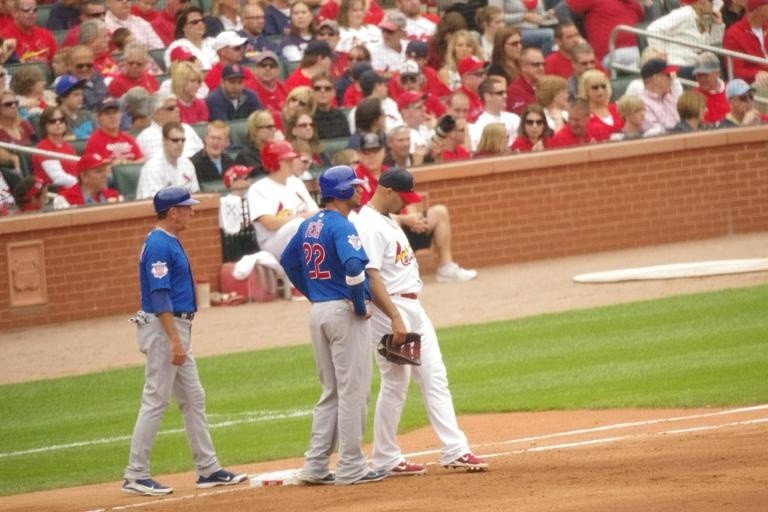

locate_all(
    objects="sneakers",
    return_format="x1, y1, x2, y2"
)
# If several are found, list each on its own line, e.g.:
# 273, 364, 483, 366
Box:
352, 468, 391, 484
195, 469, 249, 489
436, 262, 478, 282
122, 478, 174, 498
443, 454, 489, 472
314, 471, 336, 483
388, 461, 427, 477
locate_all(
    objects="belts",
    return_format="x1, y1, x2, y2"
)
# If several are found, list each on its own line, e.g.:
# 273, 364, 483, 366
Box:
402, 291, 418, 302
173, 311, 196, 322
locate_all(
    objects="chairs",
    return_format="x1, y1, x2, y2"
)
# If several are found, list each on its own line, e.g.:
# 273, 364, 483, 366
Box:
1, 0, 414, 220
413, 1, 767, 169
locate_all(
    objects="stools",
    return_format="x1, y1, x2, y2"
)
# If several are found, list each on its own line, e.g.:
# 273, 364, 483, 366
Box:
255, 257, 297, 301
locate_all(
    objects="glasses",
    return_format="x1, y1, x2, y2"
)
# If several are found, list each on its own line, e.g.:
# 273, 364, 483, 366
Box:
0, 0, 755, 143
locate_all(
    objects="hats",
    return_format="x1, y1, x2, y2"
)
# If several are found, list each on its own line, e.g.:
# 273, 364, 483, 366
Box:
224, 164, 256, 189
377, 166, 423, 206
76, 152, 113, 169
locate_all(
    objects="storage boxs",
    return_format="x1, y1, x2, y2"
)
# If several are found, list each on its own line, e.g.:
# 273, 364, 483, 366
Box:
220, 260, 279, 303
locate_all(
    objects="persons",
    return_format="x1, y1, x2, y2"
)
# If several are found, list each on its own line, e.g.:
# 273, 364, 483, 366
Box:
352, 133, 478, 285
278, 165, 391, 486
248, 141, 323, 302
121, 185, 247, 495
354, 167, 490, 475
219, 164, 286, 291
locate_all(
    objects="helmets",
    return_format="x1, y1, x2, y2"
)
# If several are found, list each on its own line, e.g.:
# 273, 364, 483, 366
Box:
260, 140, 300, 172
360, 132, 381, 151
152, 183, 201, 213
317, 164, 366, 201
